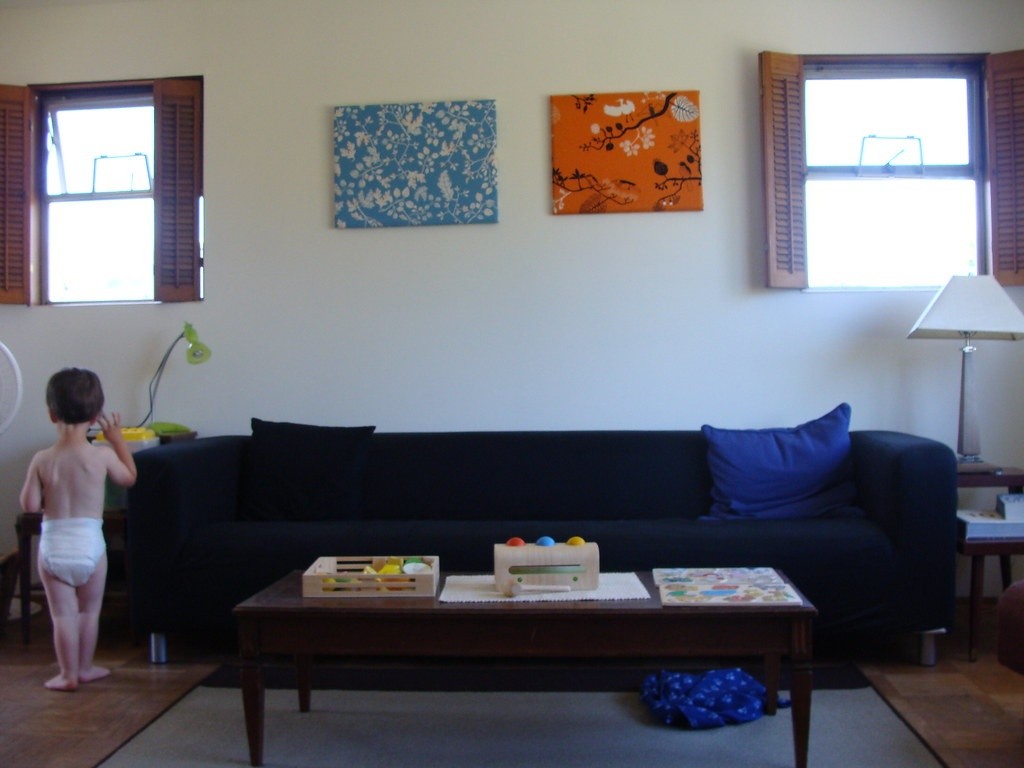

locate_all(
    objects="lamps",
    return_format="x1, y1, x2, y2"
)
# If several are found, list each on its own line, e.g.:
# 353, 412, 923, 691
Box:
136, 322, 209, 446
907, 275, 1024, 470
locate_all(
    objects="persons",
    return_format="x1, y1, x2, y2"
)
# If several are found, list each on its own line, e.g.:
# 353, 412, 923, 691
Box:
19, 367, 138, 690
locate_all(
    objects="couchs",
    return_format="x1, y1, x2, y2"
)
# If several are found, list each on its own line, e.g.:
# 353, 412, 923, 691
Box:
123, 427, 959, 666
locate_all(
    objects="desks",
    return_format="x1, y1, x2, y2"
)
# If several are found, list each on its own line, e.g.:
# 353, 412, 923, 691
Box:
954, 471, 1024, 494
15, 508, 123, 642
959, 539, 1024, 662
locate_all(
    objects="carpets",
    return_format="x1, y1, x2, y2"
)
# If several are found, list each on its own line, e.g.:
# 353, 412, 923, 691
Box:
94, 684, 949, 768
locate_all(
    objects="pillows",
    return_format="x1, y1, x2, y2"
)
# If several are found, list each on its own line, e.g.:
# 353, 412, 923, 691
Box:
696, 402, 853, 523
242, 418, 375, 523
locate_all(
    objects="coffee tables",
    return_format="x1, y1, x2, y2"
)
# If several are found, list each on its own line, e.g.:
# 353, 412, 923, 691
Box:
230, 570, 819, 768
